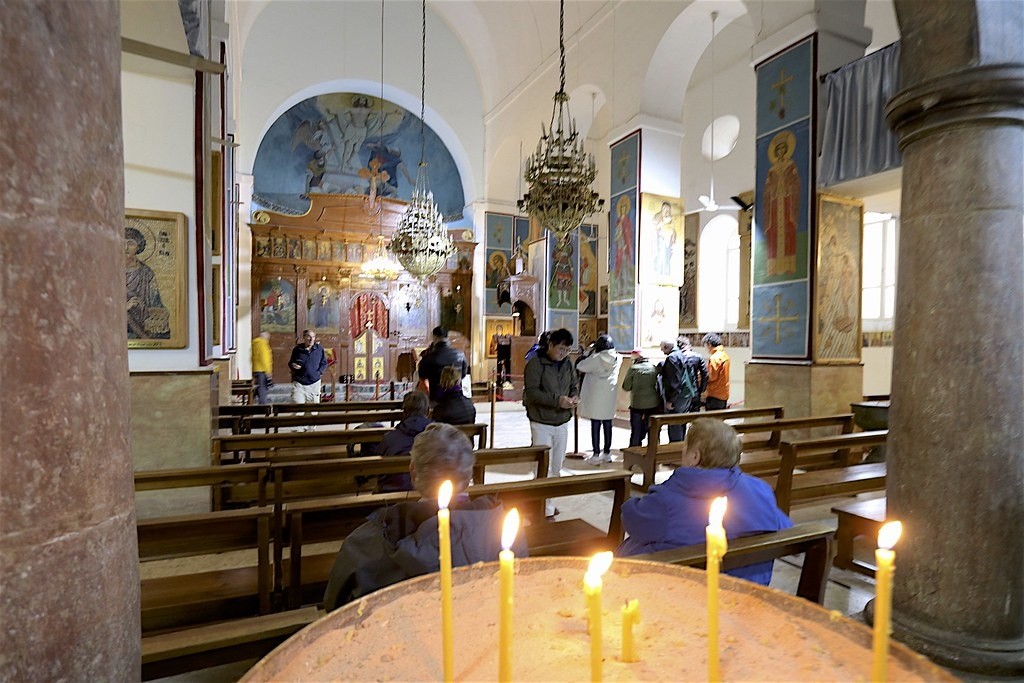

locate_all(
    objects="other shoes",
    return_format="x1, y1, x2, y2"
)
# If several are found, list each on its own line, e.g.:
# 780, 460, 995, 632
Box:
601, 453, 612, 463
583, 455, 601, 466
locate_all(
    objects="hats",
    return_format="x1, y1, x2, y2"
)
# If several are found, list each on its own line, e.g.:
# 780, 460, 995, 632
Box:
631, 348, 642, 359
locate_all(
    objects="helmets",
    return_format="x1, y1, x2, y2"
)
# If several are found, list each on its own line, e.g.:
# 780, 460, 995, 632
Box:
594, 335, 613, 350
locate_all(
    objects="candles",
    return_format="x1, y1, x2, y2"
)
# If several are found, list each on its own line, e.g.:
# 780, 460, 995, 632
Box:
706, 496, 728, 683
620, 597, 639, 661
584, 551, 613, 682
497, 506, 519, 683
437, 478, 454, 683
871, 520, 905, 683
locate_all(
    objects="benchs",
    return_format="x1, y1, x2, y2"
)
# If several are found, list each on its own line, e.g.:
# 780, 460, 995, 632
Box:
134, 392, 888, 683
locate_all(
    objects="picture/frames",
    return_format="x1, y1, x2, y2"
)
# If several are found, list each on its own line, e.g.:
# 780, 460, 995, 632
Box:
638, 193, 685, 287
122, 207, 189, 350
811, 194, 865, 365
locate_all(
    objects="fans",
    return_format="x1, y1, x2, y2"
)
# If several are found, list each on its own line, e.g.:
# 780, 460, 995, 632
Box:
680, 11, 742, 217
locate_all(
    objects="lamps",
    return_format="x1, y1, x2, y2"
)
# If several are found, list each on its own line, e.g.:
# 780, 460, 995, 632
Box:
388, 0, 461, 282
517, 0, 605, 248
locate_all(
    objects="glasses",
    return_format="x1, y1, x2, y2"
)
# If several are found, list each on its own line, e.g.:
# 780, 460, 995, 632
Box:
553, 346, 573, 356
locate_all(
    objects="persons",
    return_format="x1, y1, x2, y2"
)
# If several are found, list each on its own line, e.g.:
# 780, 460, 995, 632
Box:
289, 329, 327, 433
372, 391, 432, 494
417, 326, 468, 394
323, 423, 530, 614
431, 367, 477, 450
620, 333, 731, 452
525, 330, 551, 361
577, 335, 623, 465
523, 328, 582, 522
614, 418, 793, 587
252, 332, 273, 405
